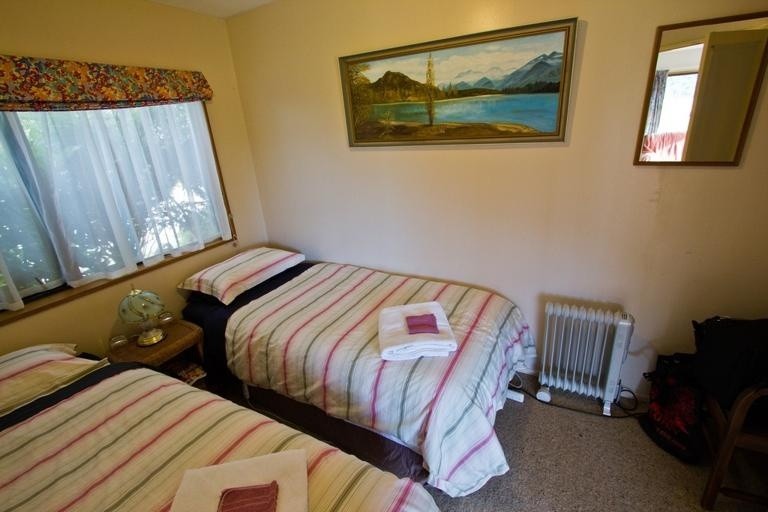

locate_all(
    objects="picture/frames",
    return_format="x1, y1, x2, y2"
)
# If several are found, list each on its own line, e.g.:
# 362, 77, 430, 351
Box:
337, 16, 579, 148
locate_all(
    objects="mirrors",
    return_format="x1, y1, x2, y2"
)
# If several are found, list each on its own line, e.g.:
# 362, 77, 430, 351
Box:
633, 12, 767, 166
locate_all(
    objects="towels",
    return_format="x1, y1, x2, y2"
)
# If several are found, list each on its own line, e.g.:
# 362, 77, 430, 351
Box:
405, 312, 439, 334
168, 448, 310, 511
377, 300, 457, 361
216, 480, 279, 511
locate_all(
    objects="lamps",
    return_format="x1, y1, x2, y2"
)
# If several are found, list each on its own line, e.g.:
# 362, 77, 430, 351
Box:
117, 281, 168, 347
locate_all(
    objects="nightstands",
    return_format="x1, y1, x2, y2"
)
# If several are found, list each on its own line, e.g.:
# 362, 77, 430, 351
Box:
107, 318, 206, 391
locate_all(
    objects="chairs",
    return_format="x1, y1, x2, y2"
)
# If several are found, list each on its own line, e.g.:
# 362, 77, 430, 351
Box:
690, 316, 768, 511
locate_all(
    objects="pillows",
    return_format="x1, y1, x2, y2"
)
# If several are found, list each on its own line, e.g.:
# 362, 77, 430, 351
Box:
176, 246, 305, 305
0, 343, 112, 432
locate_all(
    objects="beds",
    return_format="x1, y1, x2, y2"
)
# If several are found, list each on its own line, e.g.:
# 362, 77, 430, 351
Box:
0, 342, 448, 512
181, 262, 540, 500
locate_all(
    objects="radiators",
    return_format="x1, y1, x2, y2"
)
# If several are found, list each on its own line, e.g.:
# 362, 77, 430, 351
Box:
534, 299, 636, 416
